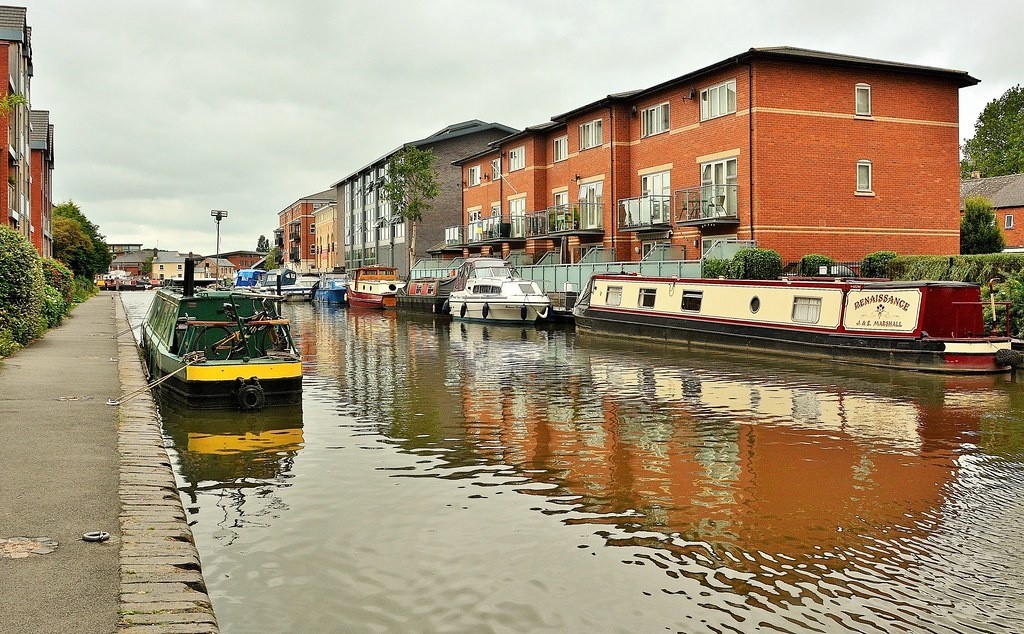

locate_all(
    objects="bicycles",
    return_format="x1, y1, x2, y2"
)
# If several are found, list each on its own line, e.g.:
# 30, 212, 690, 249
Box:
192, 295, 291, 361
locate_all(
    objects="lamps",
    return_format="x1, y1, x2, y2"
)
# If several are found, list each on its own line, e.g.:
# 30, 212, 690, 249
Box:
634, 245, 640, 253
694, 239, 698, 247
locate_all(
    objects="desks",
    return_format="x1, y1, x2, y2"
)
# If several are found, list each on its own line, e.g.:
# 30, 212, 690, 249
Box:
683, 200, 708, 220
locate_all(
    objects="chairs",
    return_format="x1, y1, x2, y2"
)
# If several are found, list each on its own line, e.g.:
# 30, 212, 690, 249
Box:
707, 195, 729, 217
679, 194, 696, 221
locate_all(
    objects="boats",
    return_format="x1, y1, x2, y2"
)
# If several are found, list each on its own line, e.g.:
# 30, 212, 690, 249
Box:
572, 270, 1015, 374
448, 257, 550, 325
143, 252, 303, 413
233, 268, 346, 304
342, 266, 407, 310
